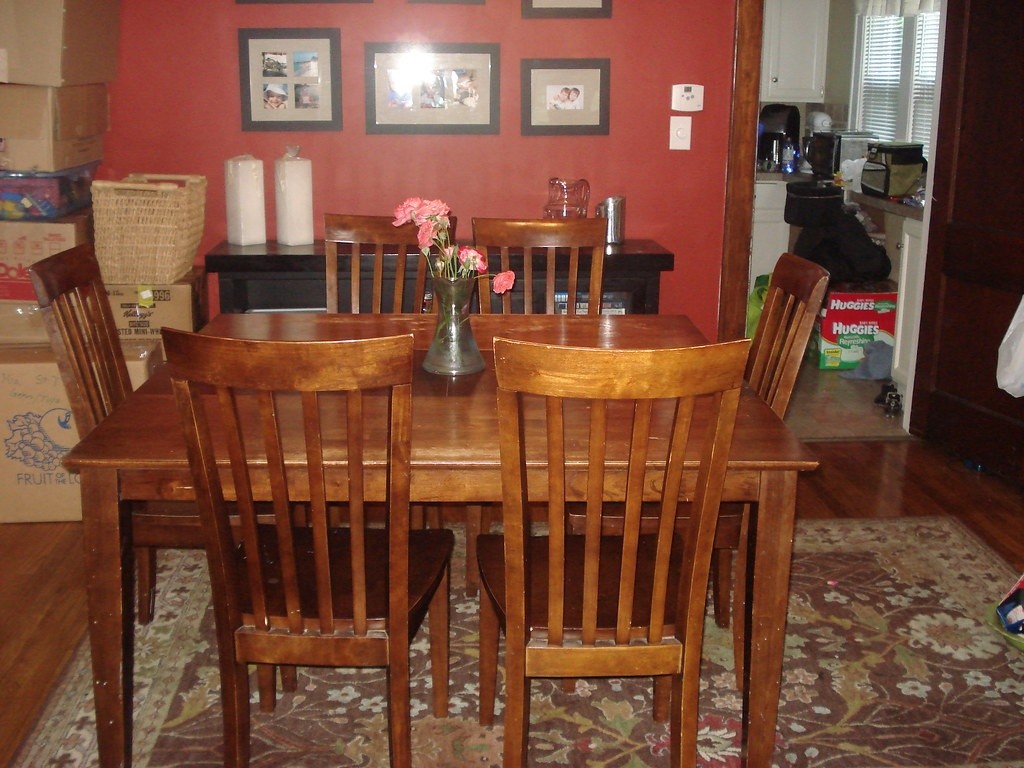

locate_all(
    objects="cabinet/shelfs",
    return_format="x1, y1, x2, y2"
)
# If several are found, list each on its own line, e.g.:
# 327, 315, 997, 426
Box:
760, 0, 829, 103
891, 218, 924, 435
749, 182, 789, 290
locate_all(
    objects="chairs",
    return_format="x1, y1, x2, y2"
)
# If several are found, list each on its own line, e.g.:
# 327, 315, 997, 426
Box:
24, 240, 297, 695
323, 211, 455, 536
471, 219, 608, 598
162, 330, 453, 764
574, 253, 828, 628
491, 335, 750, 768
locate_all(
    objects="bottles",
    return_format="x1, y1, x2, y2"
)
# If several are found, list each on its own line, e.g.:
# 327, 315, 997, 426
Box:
781, 137, 797, 173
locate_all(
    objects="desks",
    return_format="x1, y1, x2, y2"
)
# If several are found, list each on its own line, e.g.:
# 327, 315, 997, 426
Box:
61, 313, 818, 767
204, 239, 675, 312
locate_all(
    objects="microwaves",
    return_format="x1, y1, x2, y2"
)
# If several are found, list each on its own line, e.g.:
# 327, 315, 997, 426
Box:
804, 131, 879, 180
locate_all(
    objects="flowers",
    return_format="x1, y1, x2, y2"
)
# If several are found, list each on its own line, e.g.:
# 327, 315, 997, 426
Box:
395, 195, 514, 300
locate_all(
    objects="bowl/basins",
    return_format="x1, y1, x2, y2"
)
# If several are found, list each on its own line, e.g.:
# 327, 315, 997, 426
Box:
988, 601, 1024, 650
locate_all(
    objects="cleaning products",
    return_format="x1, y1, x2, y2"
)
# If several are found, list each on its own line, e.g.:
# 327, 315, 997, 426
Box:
781, 137, 800, 174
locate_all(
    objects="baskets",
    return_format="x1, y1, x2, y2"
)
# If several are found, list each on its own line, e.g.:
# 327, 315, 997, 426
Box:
90, 173, 207, 284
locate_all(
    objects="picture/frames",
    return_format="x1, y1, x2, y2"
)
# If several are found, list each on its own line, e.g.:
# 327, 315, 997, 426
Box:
239, 28, 342, 129
522, 1, 613, 19
366, 43, 503, 136
521, 58, 611, 134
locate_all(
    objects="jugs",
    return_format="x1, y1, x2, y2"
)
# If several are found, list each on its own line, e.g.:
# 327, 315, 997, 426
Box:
544, 177, 589, 220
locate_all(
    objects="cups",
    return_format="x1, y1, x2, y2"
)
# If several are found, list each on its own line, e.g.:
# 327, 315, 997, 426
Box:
596, 196, 623, 243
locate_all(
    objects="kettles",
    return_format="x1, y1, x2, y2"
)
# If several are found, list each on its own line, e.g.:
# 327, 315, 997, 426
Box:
761, 132, 783, 164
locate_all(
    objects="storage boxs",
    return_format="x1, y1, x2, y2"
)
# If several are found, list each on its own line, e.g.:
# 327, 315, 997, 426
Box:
804, 293, 901, 370
0, 0, 220, 527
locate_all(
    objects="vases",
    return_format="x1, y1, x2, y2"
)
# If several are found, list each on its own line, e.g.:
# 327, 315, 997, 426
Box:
421, 277, 487, 374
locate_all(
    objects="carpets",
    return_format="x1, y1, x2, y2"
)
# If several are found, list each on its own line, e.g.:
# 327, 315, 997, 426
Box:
5, 515, 1024, 768
